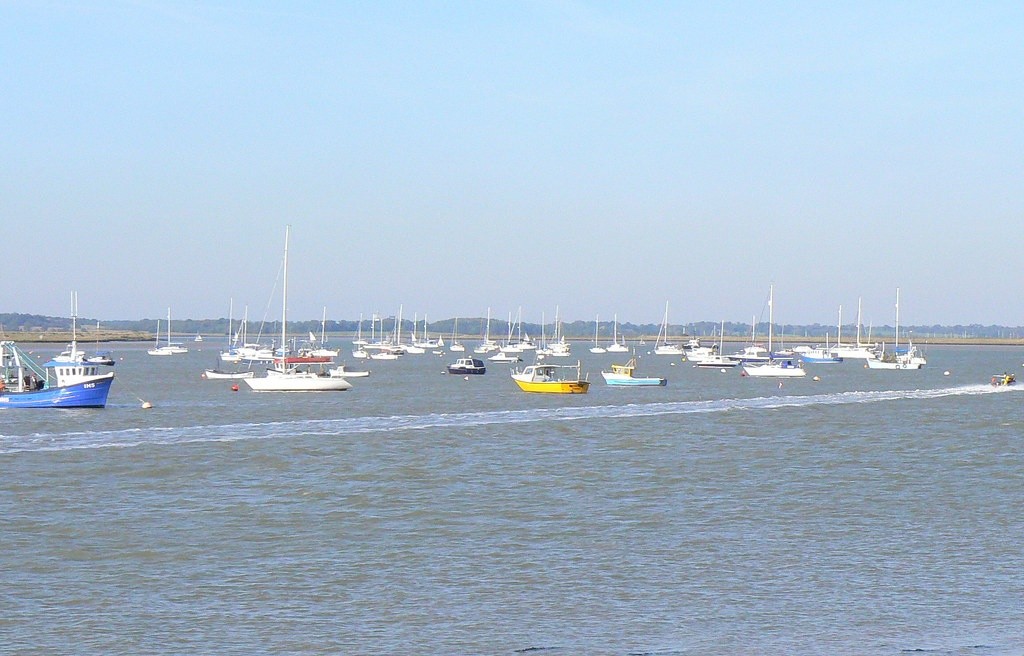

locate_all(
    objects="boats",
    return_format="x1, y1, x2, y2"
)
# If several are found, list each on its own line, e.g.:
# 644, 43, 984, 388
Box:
328, 367, 370, 377
449, 305, 572, 356
446, 358, 486, 374
86, 355, 116, 366
601, 358, 667, 386
244, 223, 354, 390
159, 307, 203, 353
147, 320, 172, 356
0, 340, 114, 408
588, 285, 806, 376
510, 359, 591, 394
487, 352, 519, 364
352, 303, 444, 360
217, 298, 341, 365
42, 344, 98, 367
792, 287, 1017, 386
204, 368, 254, 379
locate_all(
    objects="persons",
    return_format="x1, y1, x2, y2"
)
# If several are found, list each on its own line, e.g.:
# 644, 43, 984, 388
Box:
30, 373, 38, 392
546, 370, 549, 376
1004, 372, 1006, 379
